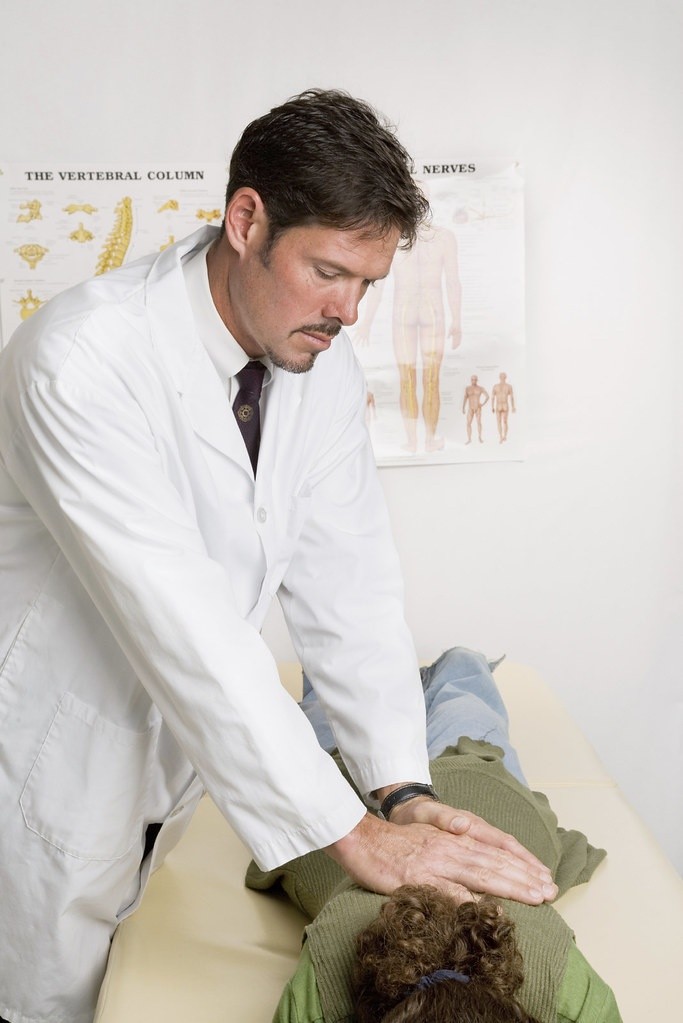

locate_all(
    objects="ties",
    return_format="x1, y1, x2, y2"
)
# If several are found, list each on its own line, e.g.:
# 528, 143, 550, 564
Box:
232, 362, 267, 483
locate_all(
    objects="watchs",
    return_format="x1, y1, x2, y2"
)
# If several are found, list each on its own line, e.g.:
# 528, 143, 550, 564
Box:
378, 783, 440, 822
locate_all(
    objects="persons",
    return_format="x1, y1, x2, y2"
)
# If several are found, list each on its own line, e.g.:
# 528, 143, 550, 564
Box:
245, 645, 625, 1023
0, 86, 561, 1023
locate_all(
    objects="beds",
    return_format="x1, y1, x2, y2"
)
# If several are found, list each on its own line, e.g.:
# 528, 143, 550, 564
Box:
95, 655, 683, 1023
